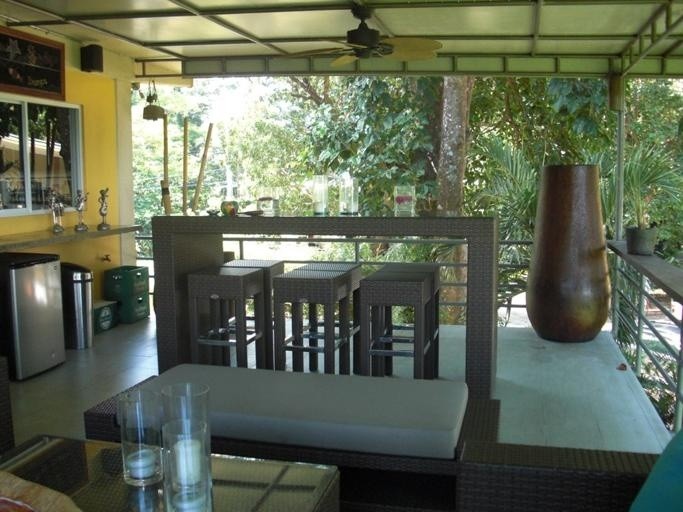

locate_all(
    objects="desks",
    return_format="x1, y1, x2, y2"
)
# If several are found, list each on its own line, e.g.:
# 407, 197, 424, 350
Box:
0, 434, 340, 511
152, 212, 498, 397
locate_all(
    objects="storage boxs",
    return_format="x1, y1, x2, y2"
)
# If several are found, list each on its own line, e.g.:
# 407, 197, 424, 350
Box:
104, 265, 149, 296
92, 298, 118, 335
104, 293, 150, 324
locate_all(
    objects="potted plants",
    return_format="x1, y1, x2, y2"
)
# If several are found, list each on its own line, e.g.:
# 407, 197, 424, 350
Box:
625, 169, 658, 255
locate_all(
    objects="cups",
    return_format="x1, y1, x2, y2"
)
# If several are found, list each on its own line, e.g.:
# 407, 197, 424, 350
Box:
162, 382, 211, 492
118, 389, 164, 487
161, 419, 212, 512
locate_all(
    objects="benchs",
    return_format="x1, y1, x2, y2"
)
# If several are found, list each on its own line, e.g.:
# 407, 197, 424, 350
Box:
116, 363, 469, 460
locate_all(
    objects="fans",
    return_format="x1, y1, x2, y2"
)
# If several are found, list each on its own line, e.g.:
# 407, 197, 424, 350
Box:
285, 6, 443, 67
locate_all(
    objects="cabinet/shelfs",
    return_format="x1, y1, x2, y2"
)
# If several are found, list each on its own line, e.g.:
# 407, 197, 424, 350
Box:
0, 224, 142, 255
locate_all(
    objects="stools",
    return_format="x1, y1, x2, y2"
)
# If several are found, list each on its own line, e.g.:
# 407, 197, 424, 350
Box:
188, 267, 266, 369
273, 271, 349, 374
376, 261, 440, 378
223, 260, 285, 370
360, 273, 432, 380
295, 264, 362, 374
